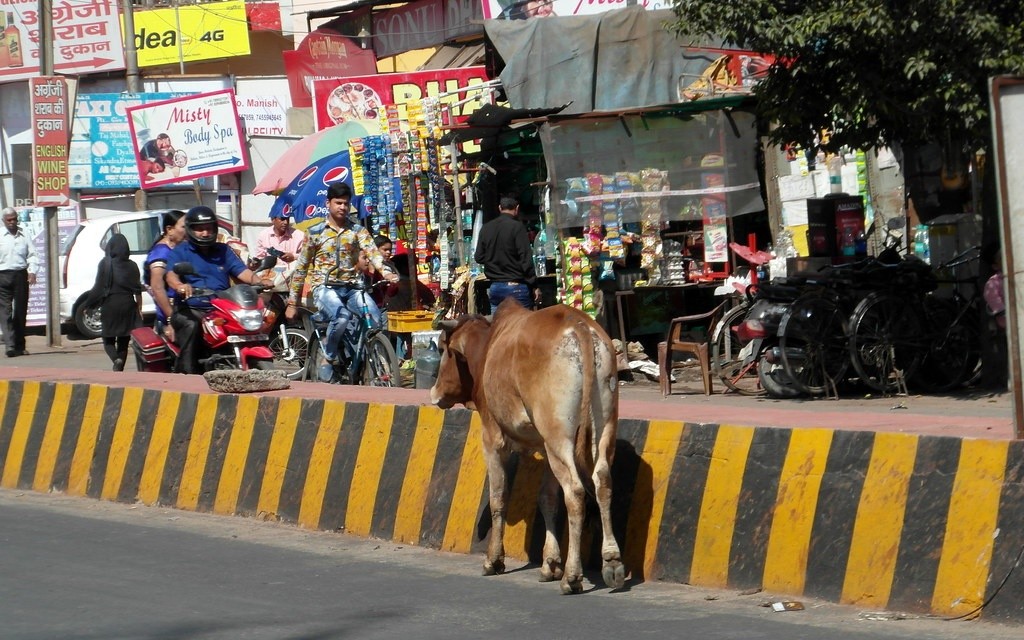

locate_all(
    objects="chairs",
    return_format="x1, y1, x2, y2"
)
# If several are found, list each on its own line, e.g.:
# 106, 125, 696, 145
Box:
658, 300, 728, 396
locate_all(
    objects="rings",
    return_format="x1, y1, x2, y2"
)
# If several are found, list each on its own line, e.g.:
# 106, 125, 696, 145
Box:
539, 302, 542, 304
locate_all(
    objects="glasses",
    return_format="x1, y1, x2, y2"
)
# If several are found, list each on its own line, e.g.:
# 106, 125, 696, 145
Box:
274, 216, 289, 221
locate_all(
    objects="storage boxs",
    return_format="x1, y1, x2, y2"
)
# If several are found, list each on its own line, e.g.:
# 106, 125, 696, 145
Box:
387, 310, 434, 333
130, 327, 169, 373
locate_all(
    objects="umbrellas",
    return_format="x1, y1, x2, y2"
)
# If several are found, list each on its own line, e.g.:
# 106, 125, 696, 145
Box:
269, 145, 405, 224
252, 121, 458, 196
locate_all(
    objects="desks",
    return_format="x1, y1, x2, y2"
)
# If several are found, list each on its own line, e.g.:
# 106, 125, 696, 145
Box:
474, 275, 558, 316
615, 281, 738, 361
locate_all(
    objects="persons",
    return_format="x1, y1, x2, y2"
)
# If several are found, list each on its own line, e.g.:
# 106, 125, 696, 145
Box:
166, 206, 274, 374
285, 183, 400, 383
429, 231, 491, 317
355, 238, 435, 366
475, 197, 543, 315
982, 248, 1008, 392
144, 209, 187, 342
84, 234, 141, 371
0, 207, 38, 353
255, 218, 311, 380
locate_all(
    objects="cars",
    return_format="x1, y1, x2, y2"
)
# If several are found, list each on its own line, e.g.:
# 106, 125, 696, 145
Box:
59, 209, 234, 340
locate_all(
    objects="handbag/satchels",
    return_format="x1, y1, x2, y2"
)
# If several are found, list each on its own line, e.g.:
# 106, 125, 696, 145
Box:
84, 256, 113, 309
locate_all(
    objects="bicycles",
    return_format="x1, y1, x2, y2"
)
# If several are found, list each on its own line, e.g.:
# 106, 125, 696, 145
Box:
710, 216, 1008, 401
268, 265, 401, 388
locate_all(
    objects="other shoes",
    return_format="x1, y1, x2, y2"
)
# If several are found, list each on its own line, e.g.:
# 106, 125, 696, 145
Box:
318, 357, 333, 382
113, 358, 124, 372
6, 349, 29, 357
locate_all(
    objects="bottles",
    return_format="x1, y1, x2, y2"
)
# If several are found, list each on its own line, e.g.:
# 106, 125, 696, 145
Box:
844, 227, 855, 255
530, 244, 536, 274
856, 227, 866, 256
536, 243, 546, 277
470, 258, 477, 276
767, 242, 773, 253
757, 265, 764, 280
915, 224, 930, 264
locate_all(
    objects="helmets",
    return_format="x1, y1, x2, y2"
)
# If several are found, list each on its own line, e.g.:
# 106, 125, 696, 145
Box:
186, 206, 219, 248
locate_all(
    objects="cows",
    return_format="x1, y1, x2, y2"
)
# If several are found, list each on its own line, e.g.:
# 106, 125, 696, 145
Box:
427, 291, 626, 595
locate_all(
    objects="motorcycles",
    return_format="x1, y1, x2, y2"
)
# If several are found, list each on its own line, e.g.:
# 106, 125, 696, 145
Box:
130, 253, 277, 371
